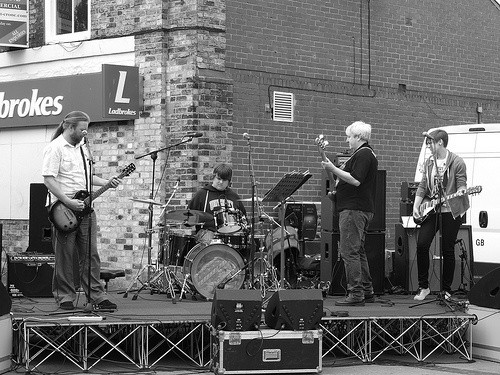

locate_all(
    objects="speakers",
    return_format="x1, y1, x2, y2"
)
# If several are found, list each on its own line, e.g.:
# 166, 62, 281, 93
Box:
265, 288, 324, 331
211, 289, 262, 331
6, 253, 56, 298
394, 223, 473, 295
321, 167, 386, 233
321, 230, 385, 295
468, 263, 500, 310
28, 183, 56, 255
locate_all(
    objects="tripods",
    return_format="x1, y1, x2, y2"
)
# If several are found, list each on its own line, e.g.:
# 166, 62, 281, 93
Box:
409, 139, 462, 312
248, 174, 292, 298
49, 137, 115, 320
122, 137, 198, 305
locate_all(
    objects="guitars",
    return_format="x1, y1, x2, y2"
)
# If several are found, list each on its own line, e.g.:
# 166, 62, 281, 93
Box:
413, 185, 482, 226
48, 162, 137, 235
314, 134, 337, 213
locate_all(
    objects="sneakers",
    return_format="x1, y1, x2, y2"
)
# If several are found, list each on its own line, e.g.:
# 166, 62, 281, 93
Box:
444, 291, 451, 299
414, 288, 430, 301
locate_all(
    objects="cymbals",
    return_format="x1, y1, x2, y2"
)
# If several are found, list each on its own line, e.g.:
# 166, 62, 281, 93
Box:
236, 196, 263, 202
129, 198, 163, 206
164, 209, 214, 223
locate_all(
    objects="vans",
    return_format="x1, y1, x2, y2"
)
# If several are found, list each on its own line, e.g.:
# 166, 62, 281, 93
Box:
413, 123, 500, 265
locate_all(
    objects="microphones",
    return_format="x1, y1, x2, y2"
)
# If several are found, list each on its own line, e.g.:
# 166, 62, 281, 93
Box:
81, 130, 88, 141
423, 132, 435, 140
243, 133, 250, 140
184, 133, 203, 137
218, 283, 225, 289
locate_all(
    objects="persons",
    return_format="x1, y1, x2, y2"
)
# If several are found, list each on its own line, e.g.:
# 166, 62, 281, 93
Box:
186, 165, 248, 244
322, 123, 379, 306
42, 112, 123, 310
413, 130, 469, 300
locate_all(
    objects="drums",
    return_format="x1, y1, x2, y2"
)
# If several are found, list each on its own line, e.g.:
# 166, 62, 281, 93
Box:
213, 232, 248, 250
215, 208, 242, 234
182, 240, 247, 301
248, 235, 268, 255
157, 227, 196, 267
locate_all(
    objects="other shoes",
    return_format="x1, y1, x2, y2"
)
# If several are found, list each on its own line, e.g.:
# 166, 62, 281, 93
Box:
364, 295, 373, 303
98, 300, 116, 309
335, 295, 365, 306
61, 301, 73, 309
